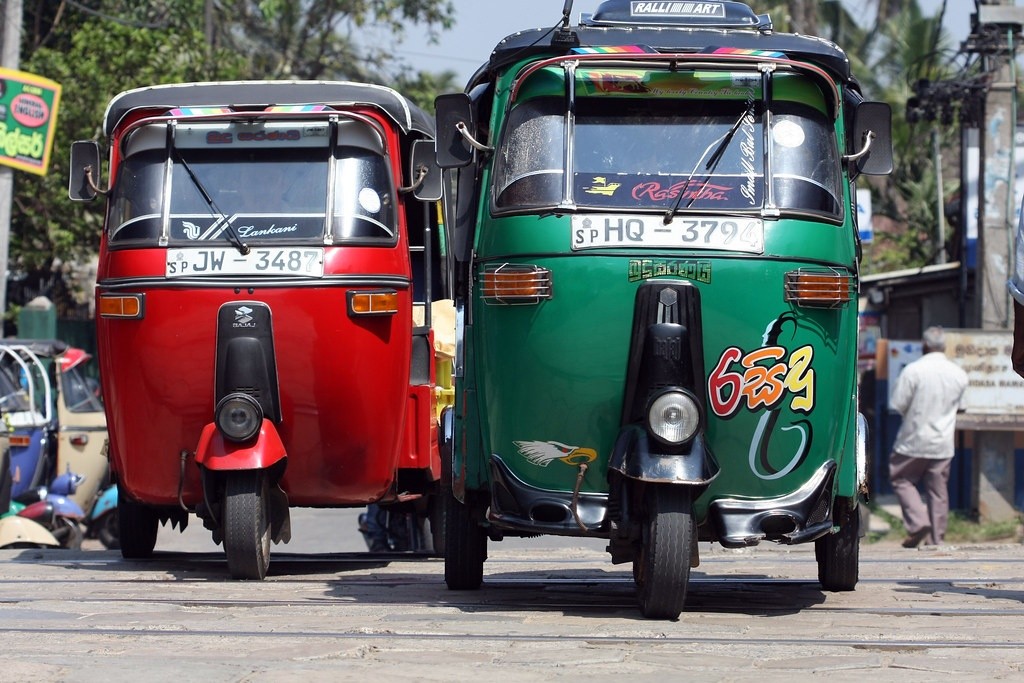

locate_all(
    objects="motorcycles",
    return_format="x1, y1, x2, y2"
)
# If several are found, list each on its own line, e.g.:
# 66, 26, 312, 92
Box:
68, 75, 454, 578
445, 0, 892, 621
0, 334, 120, 550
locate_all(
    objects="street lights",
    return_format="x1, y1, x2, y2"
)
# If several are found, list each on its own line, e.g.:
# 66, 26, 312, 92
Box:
905, 79, 963, 263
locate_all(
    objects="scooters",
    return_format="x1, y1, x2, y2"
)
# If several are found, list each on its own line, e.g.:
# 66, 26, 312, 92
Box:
359, 501, 436, 556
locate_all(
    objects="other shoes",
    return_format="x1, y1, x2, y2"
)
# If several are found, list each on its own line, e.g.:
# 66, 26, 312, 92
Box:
901, 525, 931, 548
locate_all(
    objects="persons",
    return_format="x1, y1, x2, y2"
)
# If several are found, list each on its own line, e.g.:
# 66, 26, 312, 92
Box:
1007, 196, 1024, 379
888, 327, 970, 550
